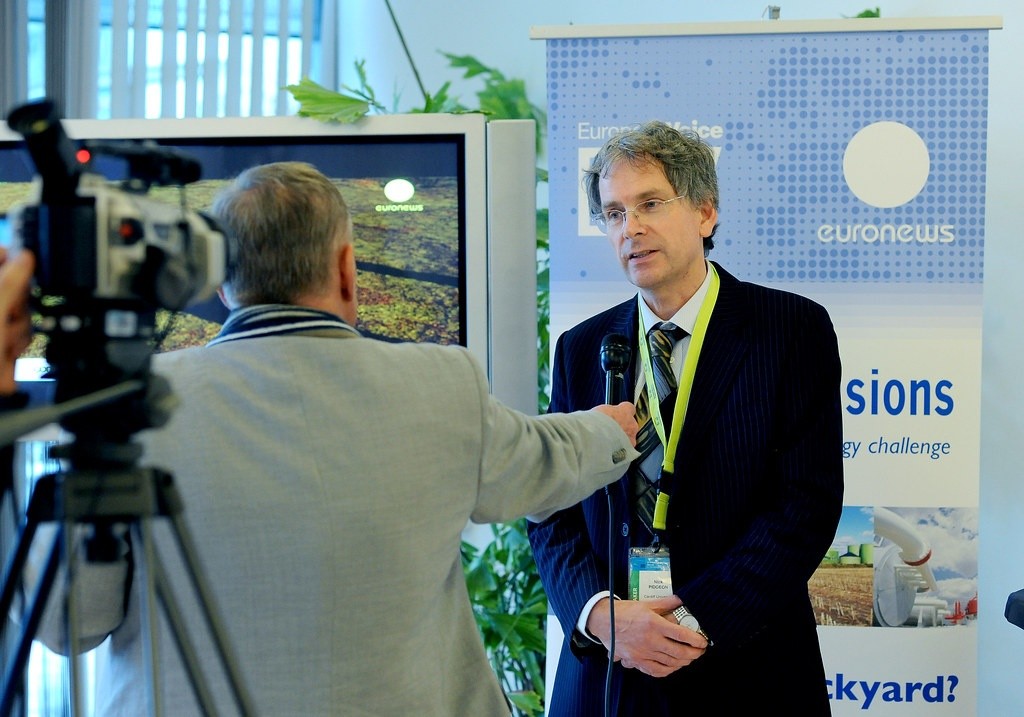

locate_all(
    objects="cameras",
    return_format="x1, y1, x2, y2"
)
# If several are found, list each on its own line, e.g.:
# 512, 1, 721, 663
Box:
7, 98, 241, 344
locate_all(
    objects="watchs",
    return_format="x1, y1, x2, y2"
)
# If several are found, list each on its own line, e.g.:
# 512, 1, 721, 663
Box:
672, 605, 708, 642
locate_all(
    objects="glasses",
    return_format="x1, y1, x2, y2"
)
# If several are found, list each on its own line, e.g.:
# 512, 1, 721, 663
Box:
593, 195, 687, 233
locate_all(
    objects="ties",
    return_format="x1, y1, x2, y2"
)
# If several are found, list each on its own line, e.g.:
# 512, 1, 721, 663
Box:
632, 326, 690, 537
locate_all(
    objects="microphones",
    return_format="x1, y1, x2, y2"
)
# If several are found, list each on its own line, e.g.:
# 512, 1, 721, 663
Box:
600, 333, 633, 405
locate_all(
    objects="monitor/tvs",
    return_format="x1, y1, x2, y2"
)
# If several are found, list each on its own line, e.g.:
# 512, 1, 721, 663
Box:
0, 121, 487, 378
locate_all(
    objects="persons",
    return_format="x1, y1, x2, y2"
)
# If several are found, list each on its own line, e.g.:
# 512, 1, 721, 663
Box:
4, 158, 641, 717
523, 118, 843, 717
0, 243, 40, 510
